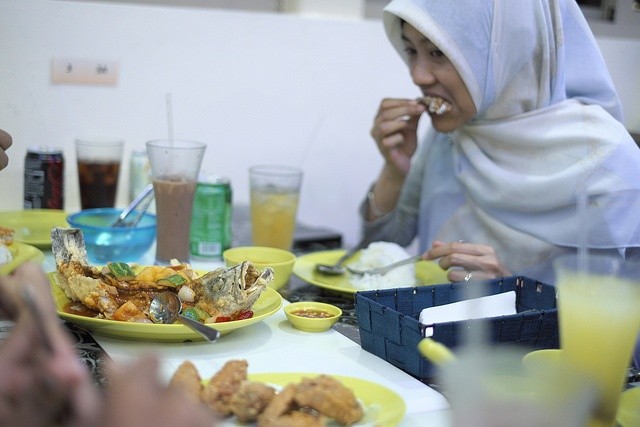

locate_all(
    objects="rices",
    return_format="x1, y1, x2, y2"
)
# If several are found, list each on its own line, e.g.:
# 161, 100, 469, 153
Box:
342, 240, 419, 291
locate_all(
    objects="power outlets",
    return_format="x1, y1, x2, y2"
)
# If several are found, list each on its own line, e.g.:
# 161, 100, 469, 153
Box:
51, 55, 89, 84
89, 57, 119, 85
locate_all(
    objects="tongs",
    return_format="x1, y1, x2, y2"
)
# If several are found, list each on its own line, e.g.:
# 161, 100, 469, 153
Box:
94, 184, 156, 261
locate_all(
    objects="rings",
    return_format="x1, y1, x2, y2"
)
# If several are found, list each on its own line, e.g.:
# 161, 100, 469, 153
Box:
462, 271, 474, 284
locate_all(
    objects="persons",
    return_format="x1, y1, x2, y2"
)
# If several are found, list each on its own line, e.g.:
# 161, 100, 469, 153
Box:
358, 0, 640, 285
0, 261, 219, 427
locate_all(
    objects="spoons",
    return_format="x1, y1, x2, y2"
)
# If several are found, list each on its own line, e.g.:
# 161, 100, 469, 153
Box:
316, 241, 366, 275
149, 291, 219, 341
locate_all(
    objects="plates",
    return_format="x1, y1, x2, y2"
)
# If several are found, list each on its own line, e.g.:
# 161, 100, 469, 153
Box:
0, 209, 73, 249
292, 248, 452, 293
195, 372, 405, 426
284, 300, 343, 331
42, 265, 283, 342
0, 241, 45, 275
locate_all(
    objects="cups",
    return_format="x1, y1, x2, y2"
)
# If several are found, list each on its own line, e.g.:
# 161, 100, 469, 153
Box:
75, 135, 122, 211
553, 253, 639, 426
145, 138, 206, 267
249, 164, 302, 251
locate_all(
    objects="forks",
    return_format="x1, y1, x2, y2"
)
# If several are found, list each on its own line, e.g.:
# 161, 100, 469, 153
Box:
344, 238, 468, 275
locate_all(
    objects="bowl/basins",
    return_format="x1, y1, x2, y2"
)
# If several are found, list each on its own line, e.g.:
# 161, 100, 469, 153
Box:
67, 207, 157, 264
222, 246, 297, 291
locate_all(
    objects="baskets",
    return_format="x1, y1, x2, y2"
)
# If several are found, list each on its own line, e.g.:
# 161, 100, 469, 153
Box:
356, 277, 558, 388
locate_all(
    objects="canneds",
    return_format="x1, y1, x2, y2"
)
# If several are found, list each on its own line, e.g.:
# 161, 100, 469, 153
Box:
21, 144, 64, 210
188, 174, 232, 262
127, 146, 158, 219
